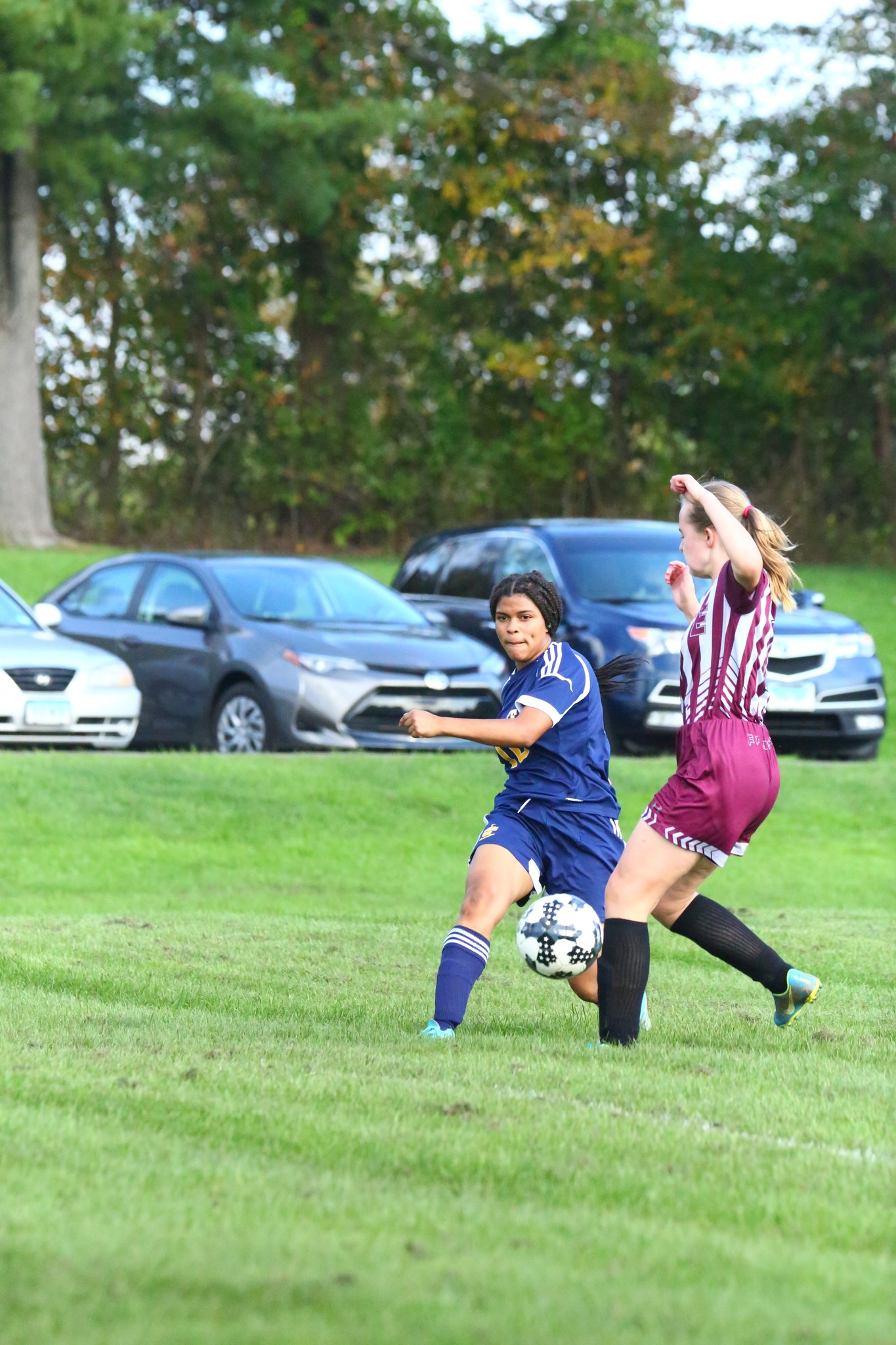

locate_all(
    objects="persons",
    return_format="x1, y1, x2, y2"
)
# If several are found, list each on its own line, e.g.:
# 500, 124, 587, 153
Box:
398, 571, 651, 1041
578, 474, 820, 1050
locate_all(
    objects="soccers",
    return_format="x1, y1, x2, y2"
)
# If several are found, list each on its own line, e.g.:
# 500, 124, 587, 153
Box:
515, 892, 604, 980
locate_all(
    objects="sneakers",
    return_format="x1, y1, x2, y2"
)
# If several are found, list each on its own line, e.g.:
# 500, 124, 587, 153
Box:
639, 990, 652, 1032
414, 1020, 455, 1040
771, 968, 823, 1029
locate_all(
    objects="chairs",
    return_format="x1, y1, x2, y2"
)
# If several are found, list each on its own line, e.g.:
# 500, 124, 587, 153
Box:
153, 579, 202, 625
255, 580, 299, 616
